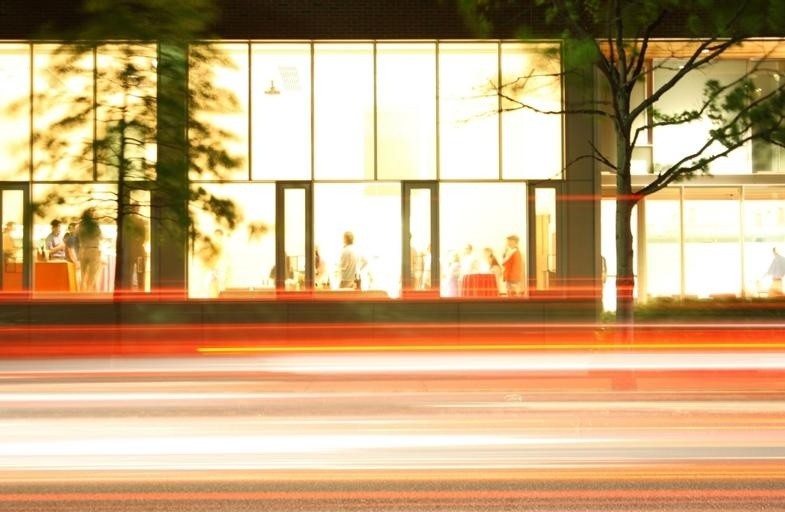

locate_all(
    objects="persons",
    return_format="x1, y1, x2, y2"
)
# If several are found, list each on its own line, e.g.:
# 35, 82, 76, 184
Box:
298, 244, 329, 289
461, 243, 479, 273
270, 253, 294, 290
335, 230, 368, 291
45, 218, 66, 259
127, 201, 148, 292
63, 222, 80, 270
447, 254, 461, 298
768, 247, 785, 294
3, 221, 23, 262
483, 247, 502, 291
501, 235, 525, 295
73, 207, 103, 291
